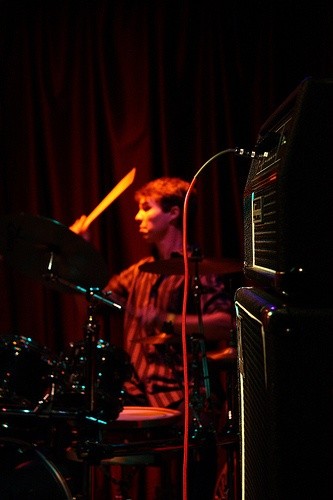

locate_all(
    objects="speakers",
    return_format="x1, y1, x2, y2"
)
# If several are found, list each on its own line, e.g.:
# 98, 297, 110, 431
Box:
235, 286, 333, 500
242, 78, 333, 302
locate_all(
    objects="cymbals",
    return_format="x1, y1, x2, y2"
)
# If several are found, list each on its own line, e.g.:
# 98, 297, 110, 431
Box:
0, 216, 106, 287
137, 255, 244, 278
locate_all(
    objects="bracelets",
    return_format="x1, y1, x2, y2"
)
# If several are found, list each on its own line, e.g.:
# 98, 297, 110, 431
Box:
158, 313, 176, 335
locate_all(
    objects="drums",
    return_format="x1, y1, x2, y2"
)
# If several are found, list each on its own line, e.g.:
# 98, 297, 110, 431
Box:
0, 434, 74, 500
93, 457, 176, 499
50, 341, 133, 430
1, 333, 60, 433
110, 405, 181, 443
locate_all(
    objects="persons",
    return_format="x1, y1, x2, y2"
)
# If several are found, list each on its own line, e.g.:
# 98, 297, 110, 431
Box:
72, 176, 236, 500
